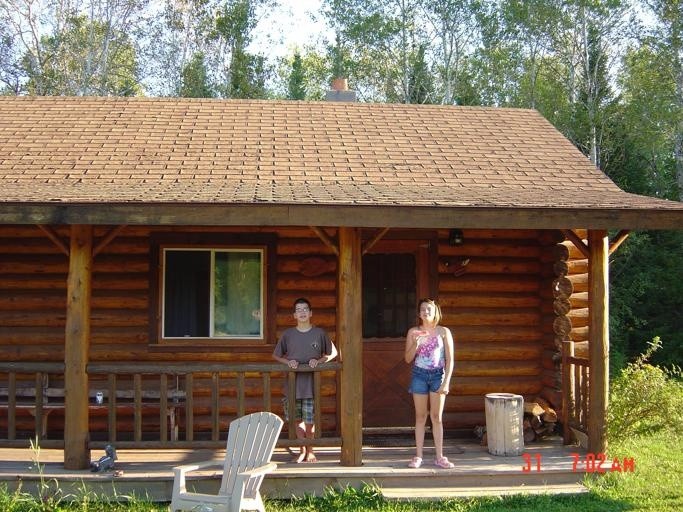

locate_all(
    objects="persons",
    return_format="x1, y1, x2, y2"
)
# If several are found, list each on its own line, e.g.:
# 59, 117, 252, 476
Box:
404, 297, 454, 470
272, 297, 338, 464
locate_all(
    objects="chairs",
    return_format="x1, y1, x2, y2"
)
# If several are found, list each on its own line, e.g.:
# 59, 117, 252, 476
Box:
169, 411, 284, 512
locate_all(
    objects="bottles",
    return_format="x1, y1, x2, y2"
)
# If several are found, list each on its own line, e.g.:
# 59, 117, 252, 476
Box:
96, 391, 104, 406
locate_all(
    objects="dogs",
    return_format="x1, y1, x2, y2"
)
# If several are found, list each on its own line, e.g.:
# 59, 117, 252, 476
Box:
90, 445, 118, 473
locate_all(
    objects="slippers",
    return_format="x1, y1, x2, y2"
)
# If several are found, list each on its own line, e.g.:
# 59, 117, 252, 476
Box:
434, 456, 454, 469
407, 455, 425, 469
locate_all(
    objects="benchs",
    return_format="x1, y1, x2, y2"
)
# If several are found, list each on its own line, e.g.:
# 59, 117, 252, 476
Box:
0, 362, 186, 441
